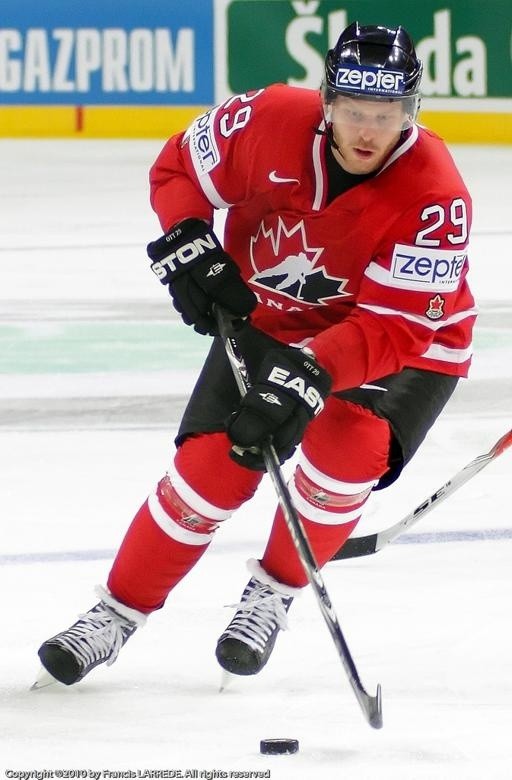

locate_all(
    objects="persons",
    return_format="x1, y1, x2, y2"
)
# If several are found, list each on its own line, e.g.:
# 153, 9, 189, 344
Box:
37, 22, 479, 686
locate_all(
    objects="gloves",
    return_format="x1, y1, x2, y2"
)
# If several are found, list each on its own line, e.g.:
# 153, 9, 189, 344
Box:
224, 343, 335, 473
145, 215, 258, 339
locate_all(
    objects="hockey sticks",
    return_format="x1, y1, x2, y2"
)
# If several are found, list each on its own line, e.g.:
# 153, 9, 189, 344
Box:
326, 427, 511, 562
213, 307, 384, 729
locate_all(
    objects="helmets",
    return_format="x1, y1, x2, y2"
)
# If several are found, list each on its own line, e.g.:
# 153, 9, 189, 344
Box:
319, 21, 424, 134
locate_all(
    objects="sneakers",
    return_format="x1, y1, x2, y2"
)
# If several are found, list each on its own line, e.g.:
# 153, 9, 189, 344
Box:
36, 597, 141, 687
213, 572, 296, 677
